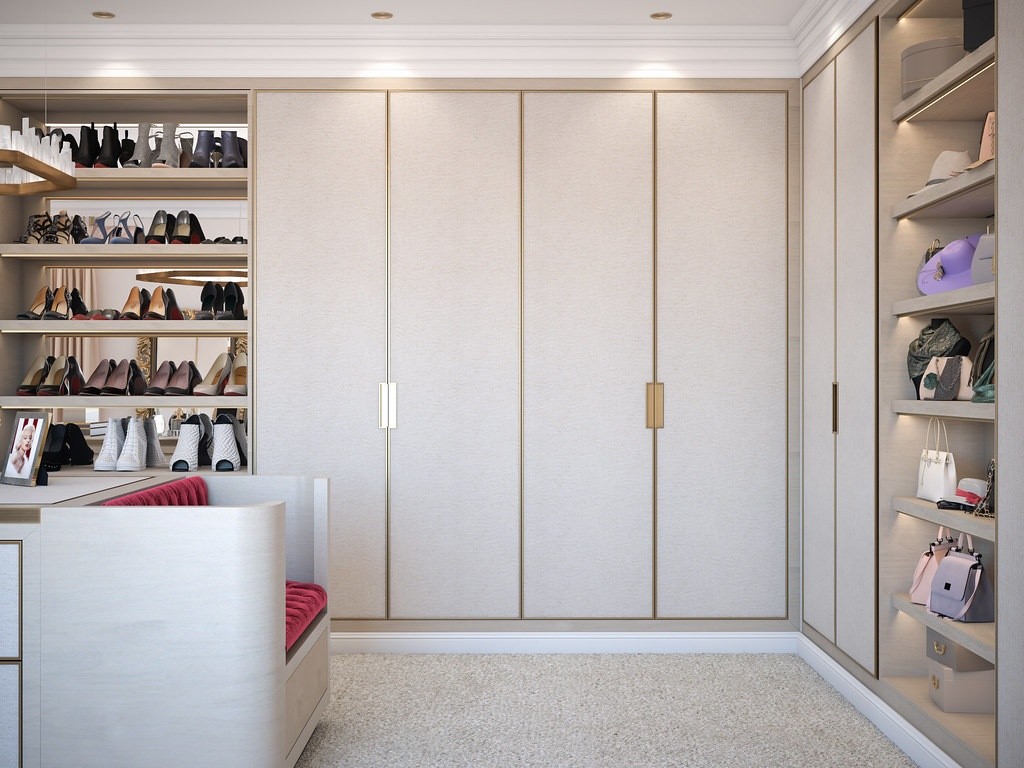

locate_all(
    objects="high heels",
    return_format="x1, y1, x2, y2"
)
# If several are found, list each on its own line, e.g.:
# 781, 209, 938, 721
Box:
11, 209, 248, 245
33, 410, 248, 472
15, 281, 246, 320
16, 352, 248, 396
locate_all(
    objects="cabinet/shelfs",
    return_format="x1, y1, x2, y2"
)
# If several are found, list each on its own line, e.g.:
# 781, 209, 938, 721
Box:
0, 86, 257, 478
802, 15, 880, 682
253, 86, 523, 621
524, 86, 788, 620
880, 0, 1002, 768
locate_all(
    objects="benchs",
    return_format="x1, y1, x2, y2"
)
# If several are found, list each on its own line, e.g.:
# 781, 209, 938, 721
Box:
39, 473, 331, 768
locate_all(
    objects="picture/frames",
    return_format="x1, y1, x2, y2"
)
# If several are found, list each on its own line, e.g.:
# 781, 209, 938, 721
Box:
0, 411, 52, 487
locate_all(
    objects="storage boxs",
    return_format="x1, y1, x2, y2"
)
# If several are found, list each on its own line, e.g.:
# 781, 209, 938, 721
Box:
925, 625, 994, 672
928, 658, 996, 715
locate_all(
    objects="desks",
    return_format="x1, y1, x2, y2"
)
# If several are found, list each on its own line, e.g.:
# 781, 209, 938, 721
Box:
0, 475, 185, 768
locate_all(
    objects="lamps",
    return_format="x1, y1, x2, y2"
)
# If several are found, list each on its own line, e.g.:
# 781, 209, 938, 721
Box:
0, 116, 76, 196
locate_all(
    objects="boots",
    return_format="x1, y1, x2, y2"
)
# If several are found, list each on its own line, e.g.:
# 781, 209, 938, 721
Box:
27, 118, 249, 169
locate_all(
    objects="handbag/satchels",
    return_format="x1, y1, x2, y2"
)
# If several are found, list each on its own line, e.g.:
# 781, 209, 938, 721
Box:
915, 417, 957, 503
918, 355, 976, 400
899, 36, 964, 100
915, 239, 947, 295
975, 460, 997, 518
972, 224, 995, 284
936, 536, 996, 624
910, 526, 958, 606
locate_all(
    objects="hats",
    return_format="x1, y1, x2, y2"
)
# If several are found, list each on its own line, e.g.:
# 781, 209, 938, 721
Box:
919, 233, 981, 295
940, 478, 987, 506
966, 112, 996, 170
906, 151, 972, 198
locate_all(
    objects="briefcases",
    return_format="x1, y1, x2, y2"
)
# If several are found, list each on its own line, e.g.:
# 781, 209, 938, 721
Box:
929, 657, 995, 713
924, 625, 994, 672
